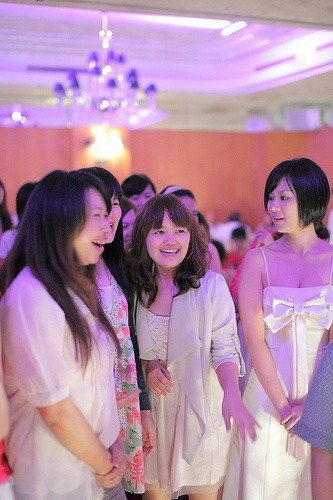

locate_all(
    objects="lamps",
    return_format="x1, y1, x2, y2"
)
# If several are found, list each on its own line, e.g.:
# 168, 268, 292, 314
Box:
53, 13, 166, 163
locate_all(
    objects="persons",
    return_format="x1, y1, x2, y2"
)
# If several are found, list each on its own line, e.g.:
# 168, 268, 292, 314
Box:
222, 158, 333, 499
0, 167, 262, 500
